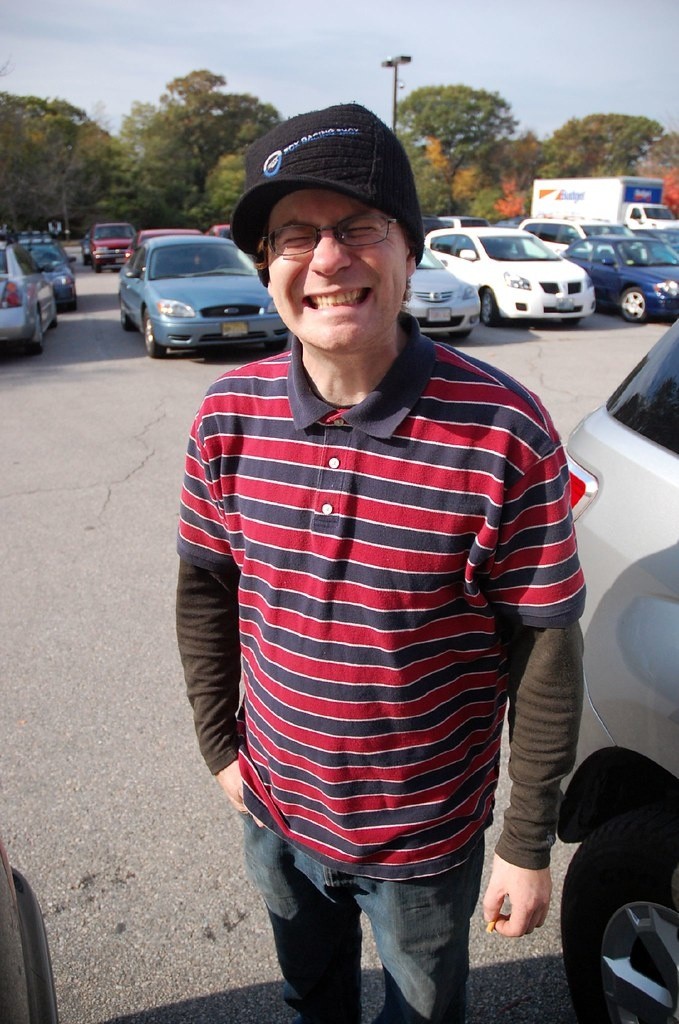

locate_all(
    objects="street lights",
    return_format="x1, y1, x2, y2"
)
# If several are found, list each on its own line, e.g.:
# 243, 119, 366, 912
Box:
381, 55, 412, 139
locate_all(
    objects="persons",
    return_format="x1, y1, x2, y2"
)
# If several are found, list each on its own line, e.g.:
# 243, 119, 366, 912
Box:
172, 104, 588, 1024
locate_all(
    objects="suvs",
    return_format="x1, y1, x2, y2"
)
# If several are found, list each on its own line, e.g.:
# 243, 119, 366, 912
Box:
90, 223, 134, 272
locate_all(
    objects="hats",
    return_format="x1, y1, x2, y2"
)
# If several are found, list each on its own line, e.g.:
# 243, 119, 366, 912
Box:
230, 103, 424, 288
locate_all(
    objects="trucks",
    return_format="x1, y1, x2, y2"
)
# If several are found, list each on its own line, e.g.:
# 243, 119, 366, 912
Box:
530, 176, 679, 230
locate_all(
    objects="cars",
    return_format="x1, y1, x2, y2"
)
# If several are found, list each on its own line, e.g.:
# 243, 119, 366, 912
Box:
81, 230, 91, 267
118, 235, 291, 358
7, 230, 54, 245
519, 310, 679, 1024
203, 224, 231, 240
632, 228, 679, 264
402, 245, 482, 340
0, 241, 59, 355
420, 215, 444, 242
123, 228, 203, 265
423, 227, 596, 328
436, 215, 490, 229
16, 240, 77, 311
556, 234, 679, 324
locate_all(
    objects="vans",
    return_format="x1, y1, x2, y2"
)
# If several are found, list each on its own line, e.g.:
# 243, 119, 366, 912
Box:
511, 218, 646, 264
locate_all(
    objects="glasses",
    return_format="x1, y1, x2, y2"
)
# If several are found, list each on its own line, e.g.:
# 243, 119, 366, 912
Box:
257, 214, 399, 259
